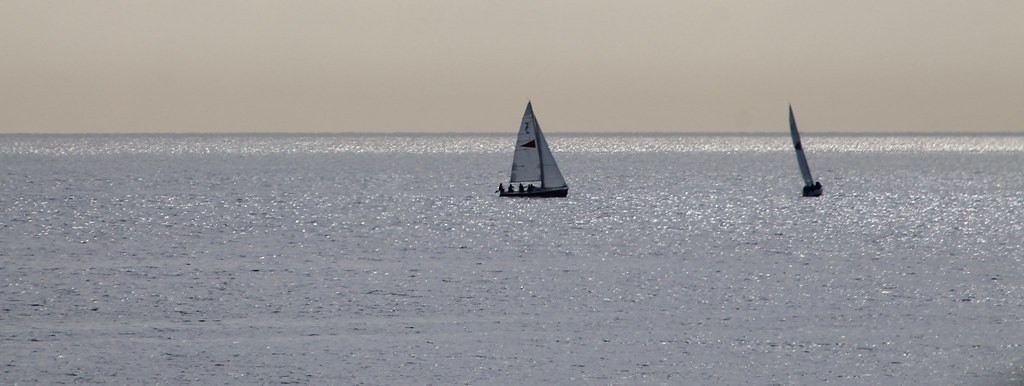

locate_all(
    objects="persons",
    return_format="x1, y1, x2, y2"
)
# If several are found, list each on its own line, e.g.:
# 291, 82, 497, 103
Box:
508, 184, 514, 192
802, 182, 821, 196
519, 183, 524, 192
527, 184, 536, 192
499, 183, 505, 192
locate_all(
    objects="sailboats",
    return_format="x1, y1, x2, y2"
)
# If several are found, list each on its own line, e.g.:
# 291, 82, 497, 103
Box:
789, 104, 823, 197
498, 100, 569, 197
